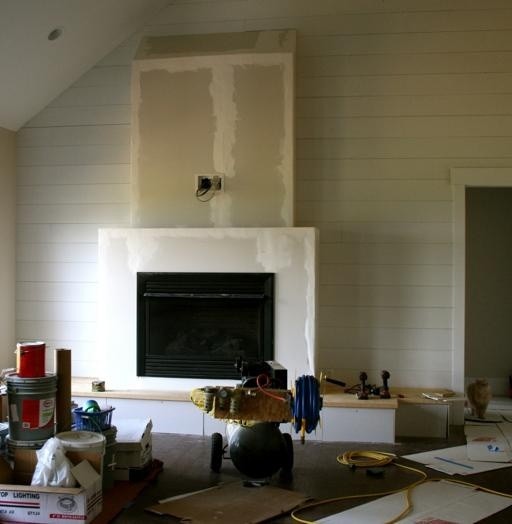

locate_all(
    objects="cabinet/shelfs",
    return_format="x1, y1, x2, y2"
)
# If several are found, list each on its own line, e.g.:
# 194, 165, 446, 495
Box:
396, 388, 451, 438
71, 383, 395, 446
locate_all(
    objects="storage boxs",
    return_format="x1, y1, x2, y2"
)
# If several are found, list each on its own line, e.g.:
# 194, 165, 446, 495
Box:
0, 448, 105, 524
112, 433, 153, 468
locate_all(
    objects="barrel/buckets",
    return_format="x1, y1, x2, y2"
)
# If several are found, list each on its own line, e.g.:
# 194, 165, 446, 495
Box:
4, 371, 58, 441
6, 433, 52, 484
53, 431, 106, 481
99, 422, 118, 492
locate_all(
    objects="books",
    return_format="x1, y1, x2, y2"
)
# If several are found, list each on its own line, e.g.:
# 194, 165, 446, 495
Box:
435, 390, 457, 397
422, 392, 457, 402
